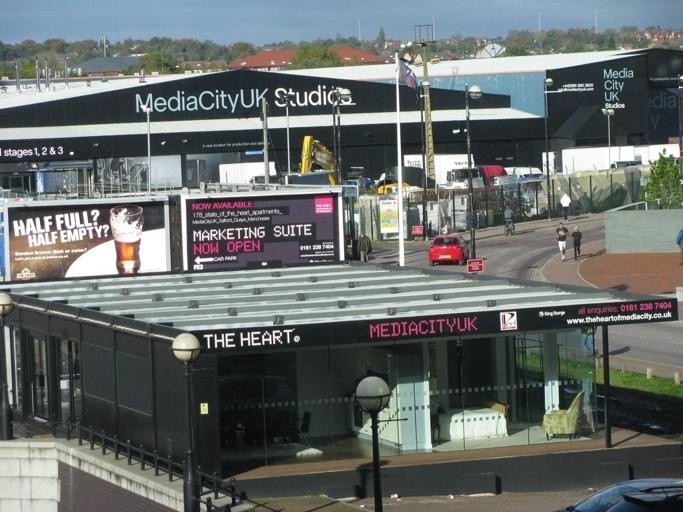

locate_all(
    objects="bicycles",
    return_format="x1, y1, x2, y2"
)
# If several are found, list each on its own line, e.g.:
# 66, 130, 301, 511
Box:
505, 221, 515, 236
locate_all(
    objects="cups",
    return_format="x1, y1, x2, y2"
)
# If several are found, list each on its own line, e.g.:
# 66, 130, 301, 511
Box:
104, 205, 143, 274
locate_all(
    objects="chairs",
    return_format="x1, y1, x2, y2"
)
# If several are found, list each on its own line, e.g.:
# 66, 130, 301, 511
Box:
543, 390, 584, 440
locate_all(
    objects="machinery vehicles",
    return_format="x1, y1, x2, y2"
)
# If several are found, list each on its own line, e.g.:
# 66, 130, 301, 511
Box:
299, 135, 437, 195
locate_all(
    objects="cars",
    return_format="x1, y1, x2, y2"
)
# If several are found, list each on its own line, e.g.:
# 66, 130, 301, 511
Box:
429, 234, 468, 265
553, 476, 682, 512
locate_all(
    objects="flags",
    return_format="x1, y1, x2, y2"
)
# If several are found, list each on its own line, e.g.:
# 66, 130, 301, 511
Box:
398, 59, 420, 106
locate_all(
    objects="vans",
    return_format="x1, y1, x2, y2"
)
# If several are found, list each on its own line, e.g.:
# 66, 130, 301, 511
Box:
479, 165, 543, 200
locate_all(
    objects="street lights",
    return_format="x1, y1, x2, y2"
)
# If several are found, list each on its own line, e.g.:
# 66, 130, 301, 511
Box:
330, 86, 351, 185
418, 81, 484, 261
543, 77, 553, 224
279, 91, 296, 175
140, 105, 151, 196
169, 332, 205, 511
601, 108, 614, 209
352, 376, 393, 511
0, 292, 16, 440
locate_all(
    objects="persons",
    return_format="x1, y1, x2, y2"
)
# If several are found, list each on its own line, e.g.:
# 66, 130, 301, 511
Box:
359, 233, 372, 262
572, 225, 582, 260
504, 204, 517, 230
560, 192, 572, 221
675, 228, 683, 265
580, 325, 597, 356
556, 222, 570, 261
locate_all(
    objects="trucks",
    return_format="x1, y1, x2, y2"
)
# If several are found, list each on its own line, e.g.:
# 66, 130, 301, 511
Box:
404, 153, 484, 199
